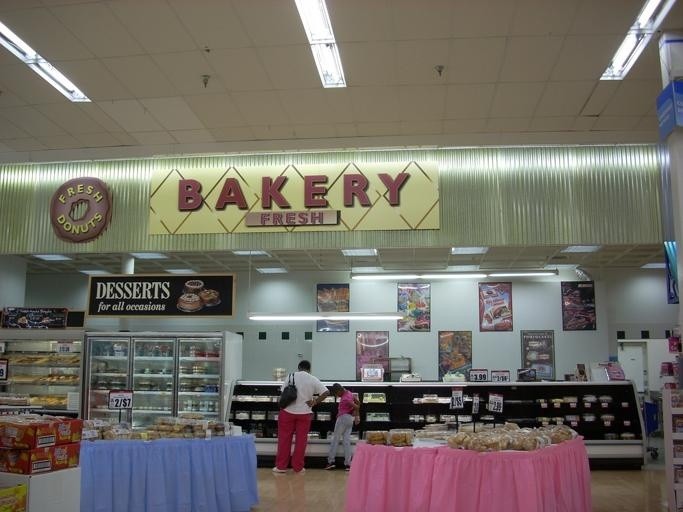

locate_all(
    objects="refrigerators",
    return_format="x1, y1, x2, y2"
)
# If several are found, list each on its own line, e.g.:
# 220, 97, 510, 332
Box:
84, 331, 243, 431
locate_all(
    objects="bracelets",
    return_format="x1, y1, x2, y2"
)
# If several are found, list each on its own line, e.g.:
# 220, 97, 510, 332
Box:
316, 398, 321, 404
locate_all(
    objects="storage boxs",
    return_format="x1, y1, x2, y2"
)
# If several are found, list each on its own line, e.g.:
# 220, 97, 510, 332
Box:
0, 414, 84, 475
0, 482, 32, 511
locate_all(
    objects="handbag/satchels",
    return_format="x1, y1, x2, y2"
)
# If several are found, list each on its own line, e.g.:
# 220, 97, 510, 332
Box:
280, 372, 297, 408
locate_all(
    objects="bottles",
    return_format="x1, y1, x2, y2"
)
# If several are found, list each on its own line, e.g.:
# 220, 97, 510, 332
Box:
182, 399, 220, 413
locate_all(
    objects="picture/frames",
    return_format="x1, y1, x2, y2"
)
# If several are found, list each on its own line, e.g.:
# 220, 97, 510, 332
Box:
315, 282, 349, 332
520, 330, 554, 380
478, 282, 514, 332
438, 330, 472, 381
396, 281, 430, 333
561, 281, 596, 331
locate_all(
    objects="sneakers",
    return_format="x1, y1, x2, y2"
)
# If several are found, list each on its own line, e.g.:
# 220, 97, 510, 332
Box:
273, 466, 286, 473
299, 467, 306, 473
324, 462, 336, 469
344, 465, 350, 471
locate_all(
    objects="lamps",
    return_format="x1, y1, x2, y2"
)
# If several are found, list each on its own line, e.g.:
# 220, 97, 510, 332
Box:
348, 269, 558, 281
244, 311, 408, 324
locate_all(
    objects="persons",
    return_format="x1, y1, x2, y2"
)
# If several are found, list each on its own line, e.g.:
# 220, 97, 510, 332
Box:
271, 359, 330, 473
324, 382, 362, 472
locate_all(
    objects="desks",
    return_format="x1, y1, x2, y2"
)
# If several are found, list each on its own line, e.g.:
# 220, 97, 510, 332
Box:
78, 422, 260, 512
344, 423, 592, 512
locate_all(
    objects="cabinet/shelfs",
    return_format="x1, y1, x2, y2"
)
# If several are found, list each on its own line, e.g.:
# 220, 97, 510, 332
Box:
224, 380, 645, 471
662, 380, 683, 512
0, 328, 84, 418
